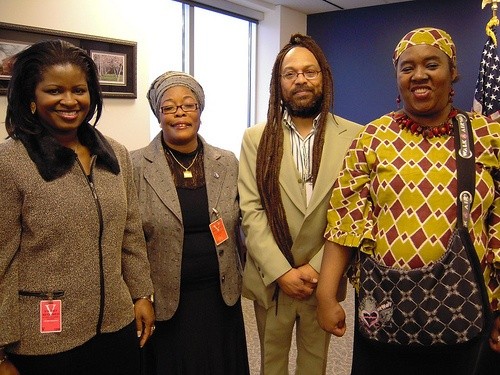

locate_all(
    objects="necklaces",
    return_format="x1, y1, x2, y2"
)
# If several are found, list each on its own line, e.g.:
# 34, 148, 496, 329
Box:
168, 149, 199, 178
393, 110, 453, 138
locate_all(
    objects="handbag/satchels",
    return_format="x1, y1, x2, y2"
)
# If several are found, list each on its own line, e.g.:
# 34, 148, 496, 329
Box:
357, 228, 492, 351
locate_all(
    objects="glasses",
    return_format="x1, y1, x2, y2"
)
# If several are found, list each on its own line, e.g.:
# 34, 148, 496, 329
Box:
281, 70, 323, 81
160, 103, 199, 114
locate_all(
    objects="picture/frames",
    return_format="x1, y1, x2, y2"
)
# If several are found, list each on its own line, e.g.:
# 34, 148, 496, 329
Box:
0, 21, 137, 100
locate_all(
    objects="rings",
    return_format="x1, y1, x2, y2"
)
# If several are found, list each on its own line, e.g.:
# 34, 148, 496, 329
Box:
152, 325, 155, 330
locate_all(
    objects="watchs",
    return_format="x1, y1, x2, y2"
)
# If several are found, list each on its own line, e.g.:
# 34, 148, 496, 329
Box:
140, 294, 154, 303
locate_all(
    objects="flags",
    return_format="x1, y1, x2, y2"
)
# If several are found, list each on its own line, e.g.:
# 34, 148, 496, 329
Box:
473, 17, 500, 122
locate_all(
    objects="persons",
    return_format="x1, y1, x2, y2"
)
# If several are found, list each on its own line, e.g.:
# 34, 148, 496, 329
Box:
236, 34, 363, 375
124, 71, 250, 375
0, 39, 155, 375
316, 26, 500, 375
